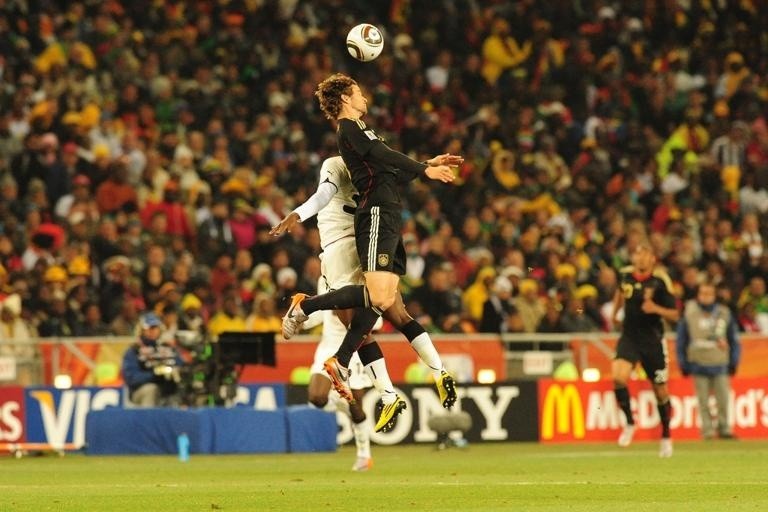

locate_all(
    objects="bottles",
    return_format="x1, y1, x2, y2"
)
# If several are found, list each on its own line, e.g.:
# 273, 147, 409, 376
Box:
176, 432, 191, 463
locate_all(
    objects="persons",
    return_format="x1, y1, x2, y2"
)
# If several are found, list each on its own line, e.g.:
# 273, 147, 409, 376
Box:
297, 275, 384, 472
269, 156, 456, 433
677, 284, 741, 439
610, 246, 679, 458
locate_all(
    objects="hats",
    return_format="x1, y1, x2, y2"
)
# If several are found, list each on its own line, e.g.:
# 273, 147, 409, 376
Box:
140, 313, 161, 329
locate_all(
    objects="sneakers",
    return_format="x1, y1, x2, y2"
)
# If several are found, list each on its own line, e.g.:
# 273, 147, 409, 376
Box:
352, 458, 374, 472
436, 372, 456, 410
375, 396, 407, 433
324, 356, 353, 402
619, 419, 639, 447
660, 438, 673, 458
282, 293, 310, 340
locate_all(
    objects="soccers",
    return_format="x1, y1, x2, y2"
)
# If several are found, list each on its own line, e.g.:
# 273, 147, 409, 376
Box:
347, 23, 384, 61
